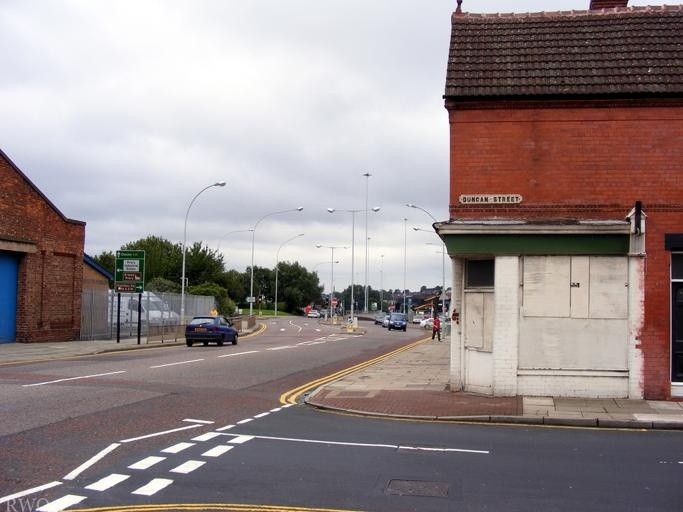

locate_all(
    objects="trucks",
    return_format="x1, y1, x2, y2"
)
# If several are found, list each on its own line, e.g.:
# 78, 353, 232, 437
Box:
108, 289, 179, 334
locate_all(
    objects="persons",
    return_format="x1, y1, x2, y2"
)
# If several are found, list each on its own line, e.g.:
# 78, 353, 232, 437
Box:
431, 314, 443, 341
210, 305, 218, 317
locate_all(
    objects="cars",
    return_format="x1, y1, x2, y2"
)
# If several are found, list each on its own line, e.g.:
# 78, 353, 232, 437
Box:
307, 303, 343, 319
372, 310, 446, 331
184, 316, 240, 347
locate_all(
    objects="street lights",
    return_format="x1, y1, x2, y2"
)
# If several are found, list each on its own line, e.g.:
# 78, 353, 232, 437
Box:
246, 207, 305, 316
315, 171, 449, 338
180, 181, 227, 325
218, 228, 254, 255
274, 233, 304, 317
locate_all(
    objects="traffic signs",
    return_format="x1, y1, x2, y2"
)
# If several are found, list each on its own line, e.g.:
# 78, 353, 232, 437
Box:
114, 250, 145, 294
